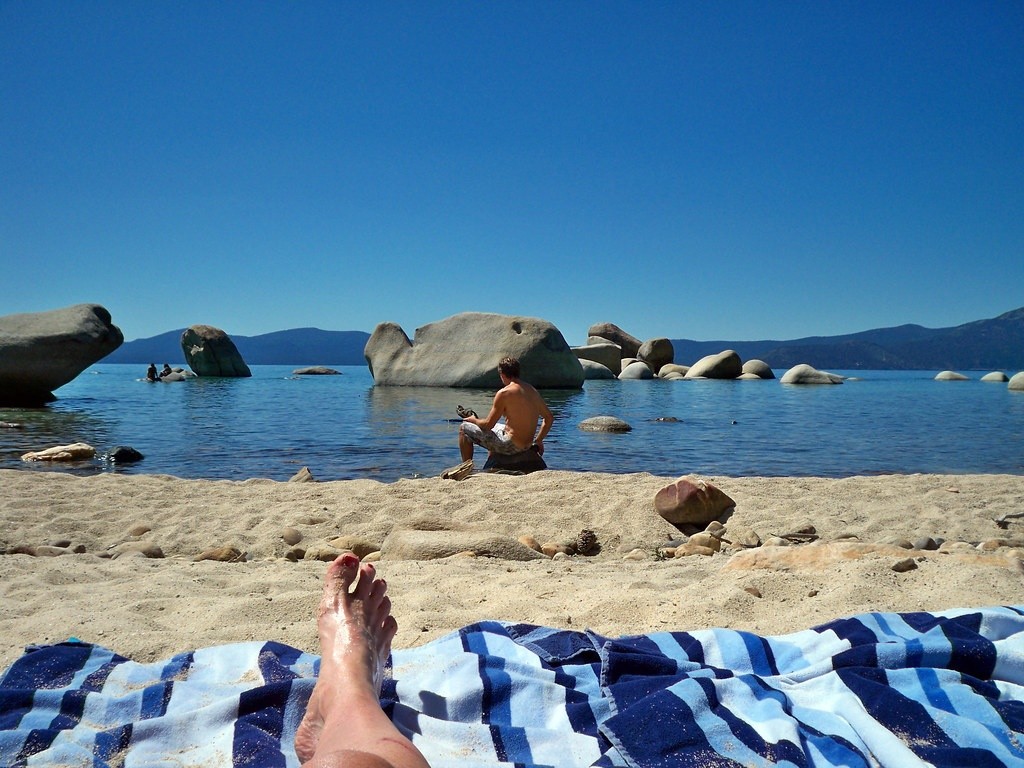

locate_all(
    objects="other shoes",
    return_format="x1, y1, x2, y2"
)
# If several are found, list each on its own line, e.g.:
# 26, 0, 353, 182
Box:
456, 405, 479, 419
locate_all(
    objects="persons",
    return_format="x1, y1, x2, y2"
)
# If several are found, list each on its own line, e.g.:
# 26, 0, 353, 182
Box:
458, 356, 554, 464
146, 363, 158, 381
294, 551, 435, 768
159, 363, 172, 378
